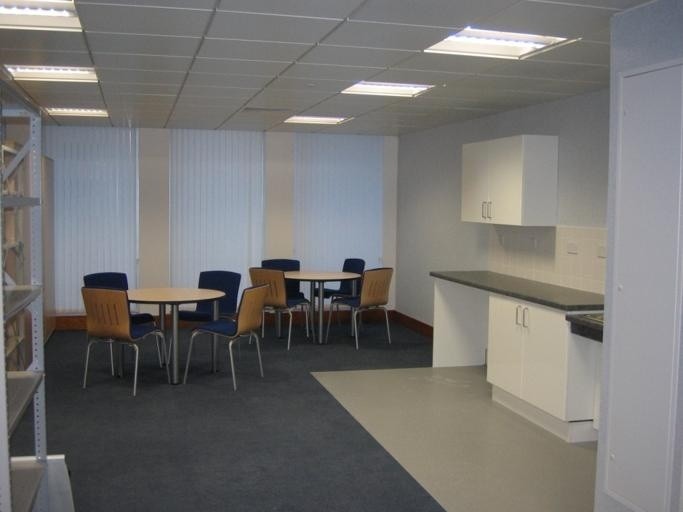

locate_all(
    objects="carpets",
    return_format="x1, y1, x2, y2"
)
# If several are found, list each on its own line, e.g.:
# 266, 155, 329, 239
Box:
11, 320, 444, 511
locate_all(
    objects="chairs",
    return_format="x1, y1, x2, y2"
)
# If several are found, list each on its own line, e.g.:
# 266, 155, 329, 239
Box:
82, 257, 393, 398
82, 270, 270, 397
249, 258, 393, 351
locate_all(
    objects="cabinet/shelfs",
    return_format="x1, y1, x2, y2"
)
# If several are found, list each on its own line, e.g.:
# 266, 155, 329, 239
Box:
458, 133, 559, 229
483, 292, 603, 445
0, 97, 46, 512
